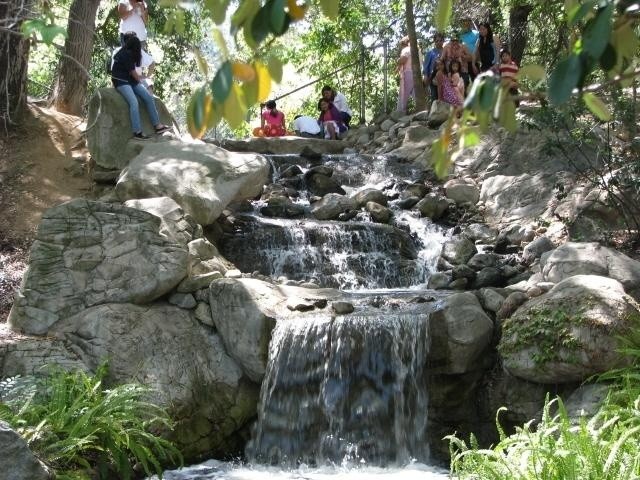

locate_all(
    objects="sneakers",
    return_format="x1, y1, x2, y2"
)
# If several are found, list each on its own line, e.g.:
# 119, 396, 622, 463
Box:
156, 125, 173, 133
135, 133, 151, 140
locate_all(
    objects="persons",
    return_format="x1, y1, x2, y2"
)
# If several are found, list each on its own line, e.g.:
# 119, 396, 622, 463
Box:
318, 99, 350, 140
113, 36, 170, 139
117, 0, 147, 48
110, 31, 155, 95
322, 86, 351, 130
294, 115, 321, 137
396, 19, 519, 108
253, 100, 286, 136
